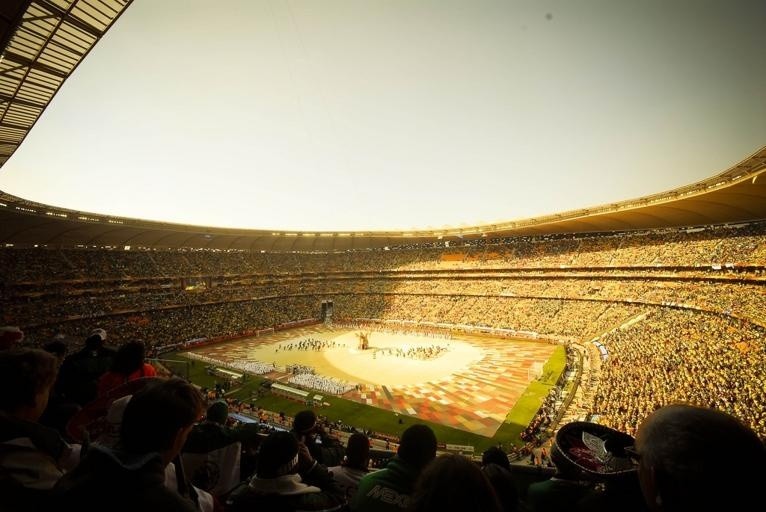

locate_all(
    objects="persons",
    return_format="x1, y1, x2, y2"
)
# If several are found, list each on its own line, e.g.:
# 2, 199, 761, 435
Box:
0, 222, 766, 512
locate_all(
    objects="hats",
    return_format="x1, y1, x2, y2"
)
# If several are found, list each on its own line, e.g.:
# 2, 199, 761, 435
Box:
87, 328, 108, 343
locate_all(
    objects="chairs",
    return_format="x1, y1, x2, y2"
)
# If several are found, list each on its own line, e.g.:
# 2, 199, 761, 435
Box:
78, 431, 198, 511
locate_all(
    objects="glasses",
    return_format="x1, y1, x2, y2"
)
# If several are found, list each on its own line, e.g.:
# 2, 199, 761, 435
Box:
623, 444, 643, 467
